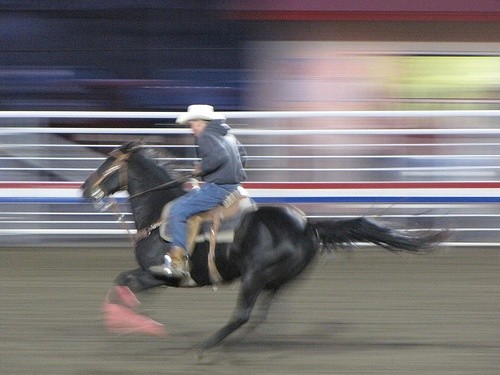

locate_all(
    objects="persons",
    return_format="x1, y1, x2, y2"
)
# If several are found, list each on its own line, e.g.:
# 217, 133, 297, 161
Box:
148, 104, 248, 278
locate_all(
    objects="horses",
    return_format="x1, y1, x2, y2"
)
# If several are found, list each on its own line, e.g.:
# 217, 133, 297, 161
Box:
80, 138, 449, 350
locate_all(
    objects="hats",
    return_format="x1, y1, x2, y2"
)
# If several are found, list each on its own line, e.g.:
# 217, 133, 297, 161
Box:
175, 104, 227, 124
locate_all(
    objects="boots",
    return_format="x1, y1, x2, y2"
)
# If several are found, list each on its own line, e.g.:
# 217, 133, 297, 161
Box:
148, 244, 187, 280
